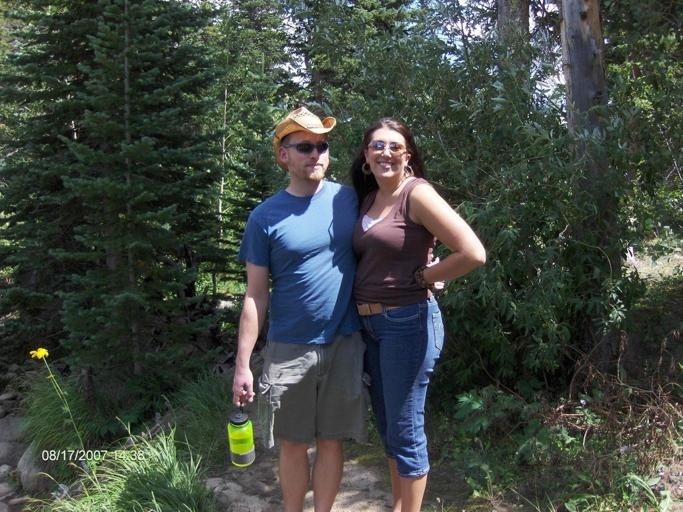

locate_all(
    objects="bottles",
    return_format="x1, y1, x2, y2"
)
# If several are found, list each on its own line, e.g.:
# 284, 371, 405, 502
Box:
227, 412, 255, 467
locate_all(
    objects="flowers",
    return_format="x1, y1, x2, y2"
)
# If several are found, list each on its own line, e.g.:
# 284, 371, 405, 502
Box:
30, 348, 105, 498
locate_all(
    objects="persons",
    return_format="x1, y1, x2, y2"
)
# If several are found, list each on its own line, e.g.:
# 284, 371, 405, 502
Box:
228, 105, 363, 512
345, 117, 487, 512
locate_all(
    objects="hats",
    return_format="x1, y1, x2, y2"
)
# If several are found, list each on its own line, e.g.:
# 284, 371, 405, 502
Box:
271, 105, 337, 170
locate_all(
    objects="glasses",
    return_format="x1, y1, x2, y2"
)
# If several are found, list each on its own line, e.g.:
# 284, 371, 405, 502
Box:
282, 142, 330, 156
367, 139, 406, 153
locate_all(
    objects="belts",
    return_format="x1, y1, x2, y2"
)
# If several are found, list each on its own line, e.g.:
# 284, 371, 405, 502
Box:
355, 302, 399, 318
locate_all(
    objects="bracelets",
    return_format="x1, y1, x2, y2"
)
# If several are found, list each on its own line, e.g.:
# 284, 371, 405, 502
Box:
413, 263, 436, 289
